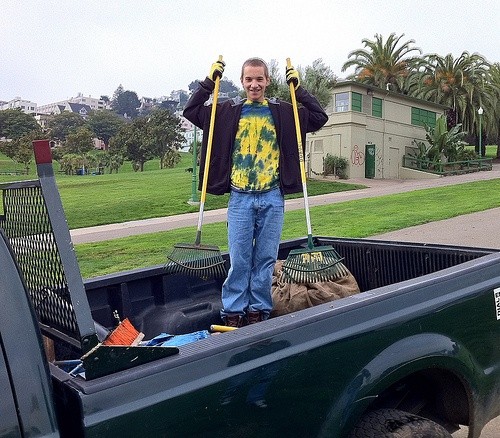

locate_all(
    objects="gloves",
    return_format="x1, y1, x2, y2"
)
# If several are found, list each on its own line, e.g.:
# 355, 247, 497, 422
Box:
286, 65, 300, 91
208, 60, 226, 81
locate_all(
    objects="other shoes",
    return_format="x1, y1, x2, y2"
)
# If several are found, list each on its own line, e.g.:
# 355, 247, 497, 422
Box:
247, 311, 259, 326
222, 315, 243, 327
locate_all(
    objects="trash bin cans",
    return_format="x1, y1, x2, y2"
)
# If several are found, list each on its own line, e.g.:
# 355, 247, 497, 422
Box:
76, 169, 83, 175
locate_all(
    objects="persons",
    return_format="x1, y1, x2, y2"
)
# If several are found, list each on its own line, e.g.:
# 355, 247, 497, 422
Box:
183, 57, 329, 327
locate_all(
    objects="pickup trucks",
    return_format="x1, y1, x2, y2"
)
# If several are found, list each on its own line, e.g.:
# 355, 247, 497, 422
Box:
0, 139, 500, 438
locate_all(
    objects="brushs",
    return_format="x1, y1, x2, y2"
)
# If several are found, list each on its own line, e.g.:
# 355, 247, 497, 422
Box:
102, 318, 145, 346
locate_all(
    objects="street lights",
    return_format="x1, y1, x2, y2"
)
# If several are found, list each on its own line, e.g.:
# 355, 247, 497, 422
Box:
478, 106, 483, 158
